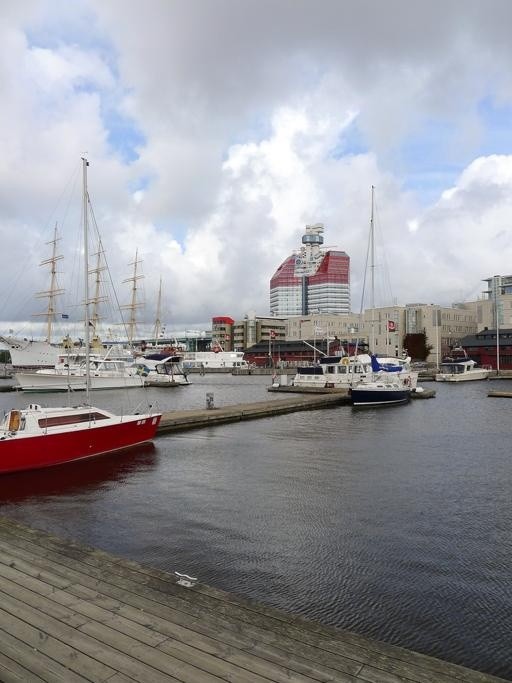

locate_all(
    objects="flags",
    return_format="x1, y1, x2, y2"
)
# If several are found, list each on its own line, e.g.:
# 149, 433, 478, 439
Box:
225, 333, 232, 340
270, 329, 276, 339
388, 319, 396, 332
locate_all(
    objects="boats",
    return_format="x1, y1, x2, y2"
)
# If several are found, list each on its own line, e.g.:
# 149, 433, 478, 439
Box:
434, 343, 488, 382
184, 349, 247, 372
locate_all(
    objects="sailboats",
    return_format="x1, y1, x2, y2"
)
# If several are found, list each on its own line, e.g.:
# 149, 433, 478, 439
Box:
1, 151, 164, 477
1, 149, 194, 394
292, 180, 418, 410
